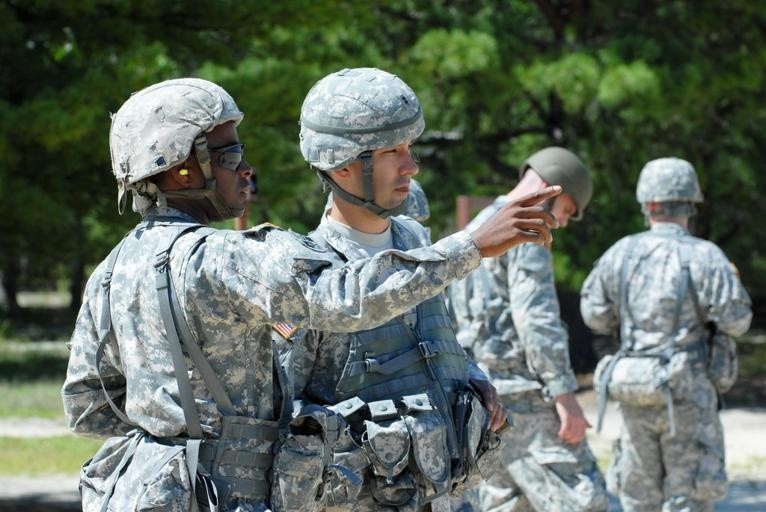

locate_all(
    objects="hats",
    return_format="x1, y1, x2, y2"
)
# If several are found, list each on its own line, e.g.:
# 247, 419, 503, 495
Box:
299, 67, 426, 172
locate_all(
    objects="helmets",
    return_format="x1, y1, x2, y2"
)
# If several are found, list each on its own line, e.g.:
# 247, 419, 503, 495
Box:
636, 156, 704, 203
520, 146, 596, 222
109, 78, 244, 185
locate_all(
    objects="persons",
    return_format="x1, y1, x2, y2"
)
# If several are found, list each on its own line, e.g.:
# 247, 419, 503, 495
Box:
446, 146, 626, 512
59, 77, 565, 512
581, 158, 758, 512
297, 66, 507, 512
323, 177, 431, 221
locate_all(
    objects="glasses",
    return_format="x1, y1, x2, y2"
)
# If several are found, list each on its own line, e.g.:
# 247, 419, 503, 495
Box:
206, 142, 245, 171
